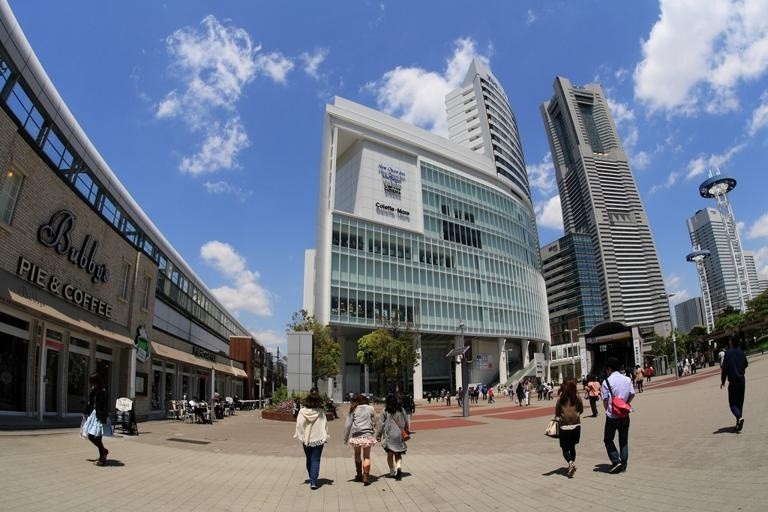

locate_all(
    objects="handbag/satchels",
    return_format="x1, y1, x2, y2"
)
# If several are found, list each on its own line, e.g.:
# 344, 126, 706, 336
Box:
584, 392, 589, 399
612, 396, 632, 418
400, 427, 410, 441
544, 420, 560, 438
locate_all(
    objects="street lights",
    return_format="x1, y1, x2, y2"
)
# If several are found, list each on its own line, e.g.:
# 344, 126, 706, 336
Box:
564, 328, 578, 380
659, 292, 680, 380
753, 337, 757, 347
501, 349, 513, 384
456, 322, 469, 417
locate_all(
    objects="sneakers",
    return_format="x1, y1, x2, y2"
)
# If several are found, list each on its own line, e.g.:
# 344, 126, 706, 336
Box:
95, 449, 108, 466
390, 471, 396, 478
562, 464, 576, 477
395, 467, 401, 481
735, 417, 744, 433
608, 461, 626, 474
311, 483, 316, 488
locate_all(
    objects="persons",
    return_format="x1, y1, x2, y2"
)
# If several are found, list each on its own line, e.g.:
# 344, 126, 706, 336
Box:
292, 398, 302, 416
152, 385, 160, 410
326, 398, 339, 418
556, 377, 584, 477
293, 394, 330, 489
582, 369, 606, 417
344, 390, 415, 404
344, 395, 377, 485
424, 376, 554, 407
167, 389, 238, 424
262, 390, 273, 408
79, 372, 112, 466
600, 358, 635, 473
621, 363, 653, 392
720, 337, 748, 431
375, 392, 409, 479
677, 348, 725, 376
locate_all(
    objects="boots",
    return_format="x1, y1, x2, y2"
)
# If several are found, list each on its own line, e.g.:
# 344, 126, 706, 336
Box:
355, 460, 362, 482
363, 463, 370, 484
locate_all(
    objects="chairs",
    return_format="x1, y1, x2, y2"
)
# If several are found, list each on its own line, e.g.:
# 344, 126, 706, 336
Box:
165, 399, 266, 425
